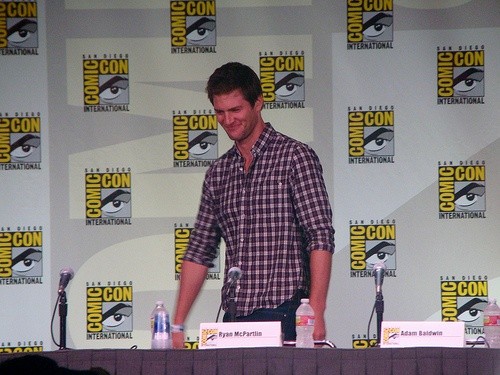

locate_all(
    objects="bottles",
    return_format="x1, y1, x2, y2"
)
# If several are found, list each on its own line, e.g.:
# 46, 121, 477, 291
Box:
484, 298, 500, 348
152, 302, 172, 349
295, 298, 315, 348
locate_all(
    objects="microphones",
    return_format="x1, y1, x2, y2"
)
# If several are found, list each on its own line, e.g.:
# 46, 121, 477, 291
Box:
225, 267, 242, 289
373, 262, 386, 300
58, 266, 75, 294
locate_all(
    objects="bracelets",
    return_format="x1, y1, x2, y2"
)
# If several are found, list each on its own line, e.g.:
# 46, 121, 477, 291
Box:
171, 324, 185, 332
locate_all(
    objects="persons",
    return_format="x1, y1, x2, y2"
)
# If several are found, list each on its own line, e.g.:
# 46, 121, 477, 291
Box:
169, 62, 336, 349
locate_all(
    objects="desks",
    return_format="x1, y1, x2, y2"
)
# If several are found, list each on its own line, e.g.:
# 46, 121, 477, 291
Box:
0, 347, 500, 375
